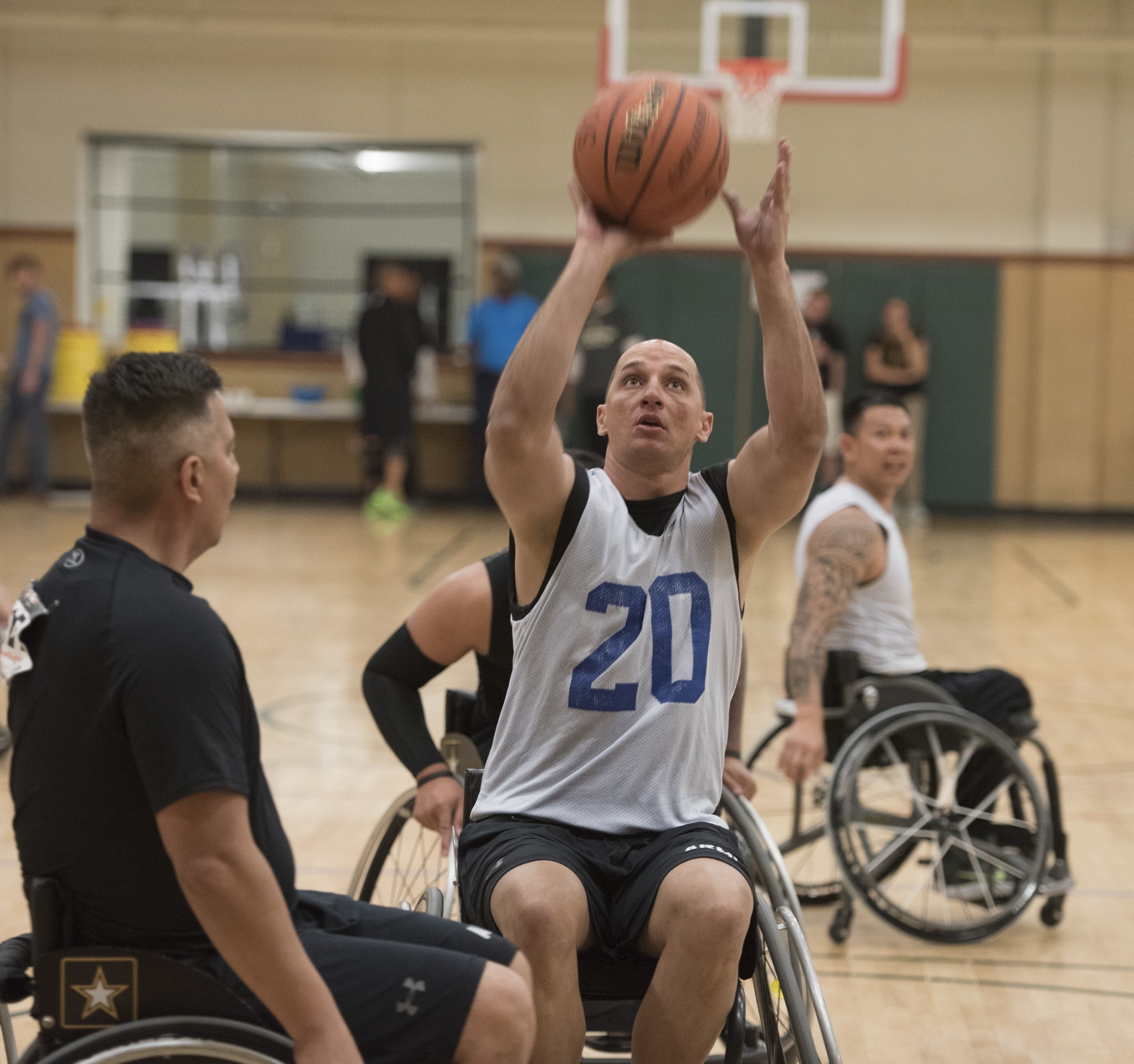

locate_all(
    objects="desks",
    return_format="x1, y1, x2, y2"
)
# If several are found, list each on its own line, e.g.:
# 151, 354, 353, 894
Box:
220, 390, 478, 497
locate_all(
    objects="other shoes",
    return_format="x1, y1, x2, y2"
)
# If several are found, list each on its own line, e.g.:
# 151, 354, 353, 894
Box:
362, 488, 403, 516
952, 866, 1010, 884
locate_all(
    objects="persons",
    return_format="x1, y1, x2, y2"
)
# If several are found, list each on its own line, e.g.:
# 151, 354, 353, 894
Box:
0, 351, 536, 1064
0, 250, 59, 498
339, 135, 1040, 1064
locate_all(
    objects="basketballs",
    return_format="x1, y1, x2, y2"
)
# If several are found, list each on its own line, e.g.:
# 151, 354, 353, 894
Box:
573, 77, 732, 229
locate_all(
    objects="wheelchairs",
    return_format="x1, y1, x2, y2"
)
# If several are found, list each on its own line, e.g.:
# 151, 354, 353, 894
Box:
0, 931, 299, 1064
721, 676, 1076, 946
399, 768, 843, 1064
346, 688, 809, 1063
